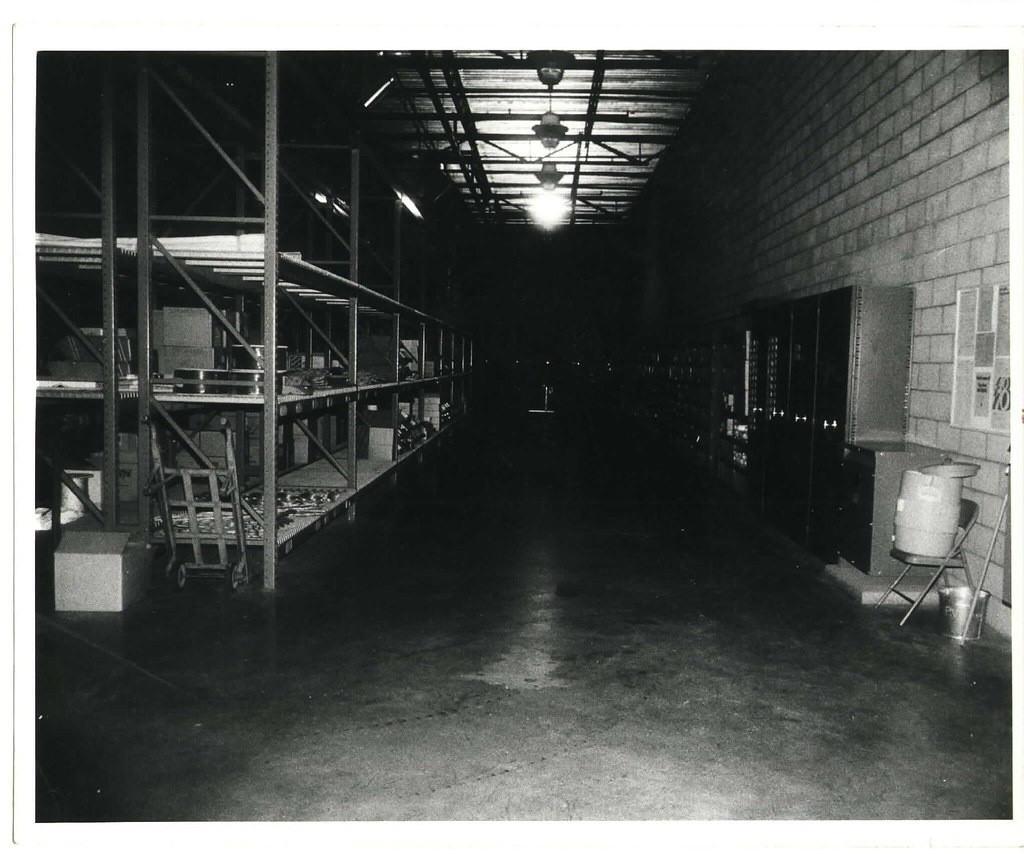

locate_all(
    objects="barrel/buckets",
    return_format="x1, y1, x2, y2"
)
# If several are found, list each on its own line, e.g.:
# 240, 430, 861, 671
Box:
938, 586, 992, 640
891, 470, 964, 558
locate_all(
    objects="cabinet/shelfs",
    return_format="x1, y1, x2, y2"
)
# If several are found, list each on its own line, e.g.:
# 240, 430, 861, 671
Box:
639, 282, 950, 579
36, 52, 486, 601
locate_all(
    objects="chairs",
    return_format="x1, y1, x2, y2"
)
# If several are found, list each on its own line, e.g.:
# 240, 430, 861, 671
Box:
874, 497, 978, 627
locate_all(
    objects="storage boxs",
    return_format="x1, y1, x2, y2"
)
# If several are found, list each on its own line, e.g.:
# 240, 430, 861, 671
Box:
45, 306, 452, 614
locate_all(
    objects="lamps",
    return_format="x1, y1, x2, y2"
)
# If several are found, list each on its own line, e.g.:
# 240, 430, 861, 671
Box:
532, 101, 569, 148
525, 50, 577, 85
534, 161, 566, 191
